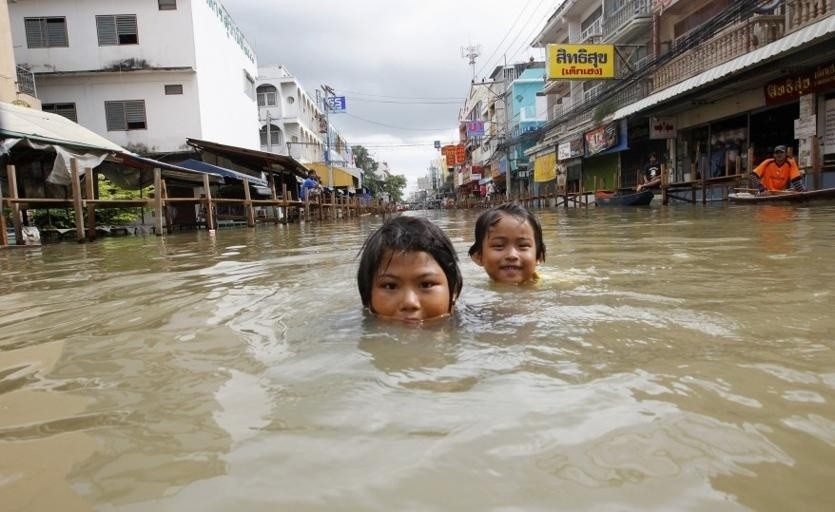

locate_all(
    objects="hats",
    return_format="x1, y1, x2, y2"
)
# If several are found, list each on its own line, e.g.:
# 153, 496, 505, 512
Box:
774, 145, 785, 153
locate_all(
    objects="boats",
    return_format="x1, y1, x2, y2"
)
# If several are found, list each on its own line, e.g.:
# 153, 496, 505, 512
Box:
595, 189, 654, 208
728, 188, 835, 207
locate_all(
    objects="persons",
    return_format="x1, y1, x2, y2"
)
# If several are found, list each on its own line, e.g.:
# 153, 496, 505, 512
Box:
466, 200, 547, 286
636, 154, 661, 192
487, 180, 499, 196
300, 169, 321, 202
356, 213, 464, 324
748, 143, 808, 196
